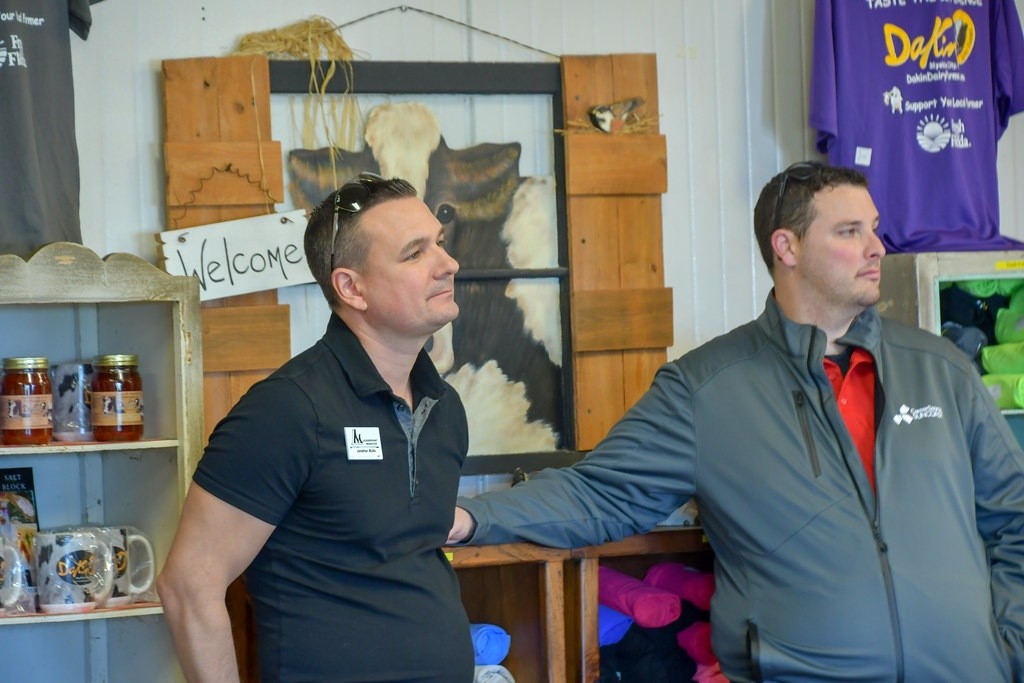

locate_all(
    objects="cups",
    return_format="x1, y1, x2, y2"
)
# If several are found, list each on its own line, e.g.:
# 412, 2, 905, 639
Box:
33, 529, 115, 613
0, 538, 25, 620
48, 360, 95, 442
89, 524, 155, 607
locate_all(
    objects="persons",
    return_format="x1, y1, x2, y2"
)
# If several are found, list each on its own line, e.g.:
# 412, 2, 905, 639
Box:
444, 156, 1024, 683
153, 178, 474, 683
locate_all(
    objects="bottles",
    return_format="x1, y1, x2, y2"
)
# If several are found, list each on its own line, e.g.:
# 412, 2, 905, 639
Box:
92, 354, 143, 442
0, 358, 52, 445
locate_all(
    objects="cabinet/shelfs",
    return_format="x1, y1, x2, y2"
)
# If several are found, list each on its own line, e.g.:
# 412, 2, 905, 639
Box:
877, 250, 1023, 416
0, 242, 204, 683
225, 528, 713, 683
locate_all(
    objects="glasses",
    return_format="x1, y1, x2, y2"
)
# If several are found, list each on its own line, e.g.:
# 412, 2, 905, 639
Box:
769, 161, 817, 229
330, 172, 384, 273
